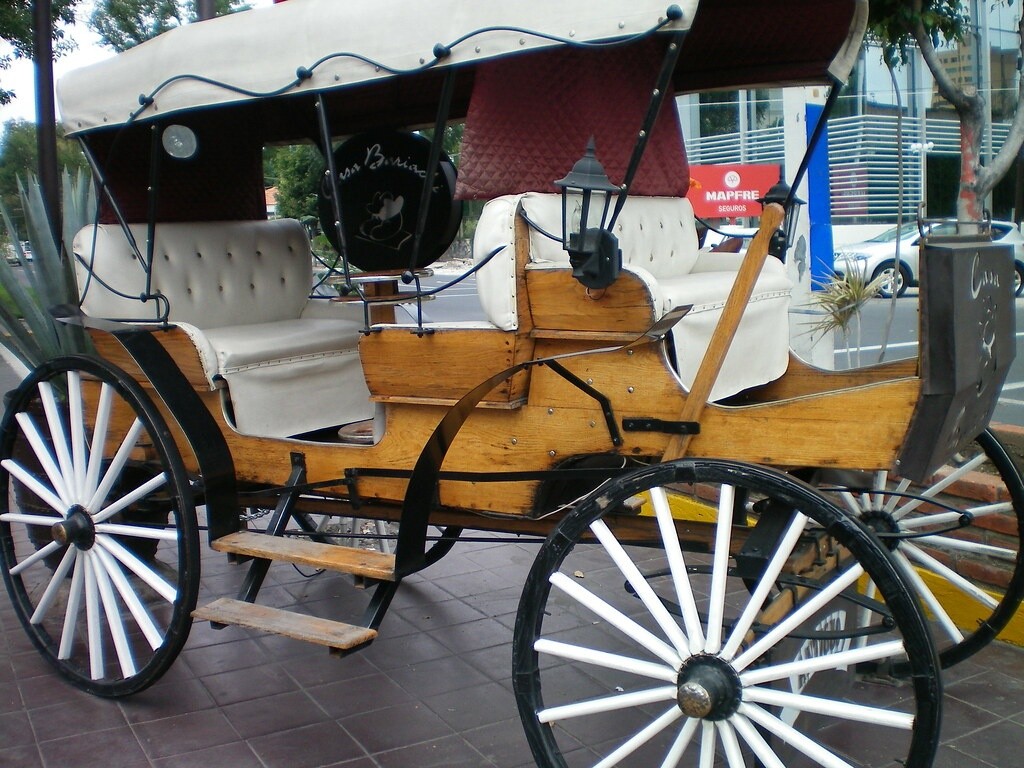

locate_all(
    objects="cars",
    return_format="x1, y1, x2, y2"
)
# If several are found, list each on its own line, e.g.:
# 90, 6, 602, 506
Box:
5, 240, 33, 267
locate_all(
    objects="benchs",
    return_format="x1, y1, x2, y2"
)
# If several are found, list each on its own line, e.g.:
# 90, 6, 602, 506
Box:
453, 60, 790, 403
71, 217, 375, 438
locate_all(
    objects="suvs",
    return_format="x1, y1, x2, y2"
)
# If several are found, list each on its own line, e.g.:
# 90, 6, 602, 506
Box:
832, 218, 1024, 299
